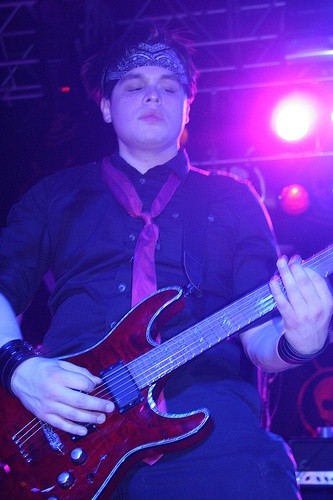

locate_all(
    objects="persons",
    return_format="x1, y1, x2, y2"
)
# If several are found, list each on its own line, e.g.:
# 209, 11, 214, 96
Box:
0, 19, 333, 500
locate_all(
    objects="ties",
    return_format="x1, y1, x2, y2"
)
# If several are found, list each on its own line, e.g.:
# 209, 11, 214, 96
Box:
102, 157, 181, 465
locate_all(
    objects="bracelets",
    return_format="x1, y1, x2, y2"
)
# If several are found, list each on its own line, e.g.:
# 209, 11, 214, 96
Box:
0, 339, 36, 399
278, 334, 330, 364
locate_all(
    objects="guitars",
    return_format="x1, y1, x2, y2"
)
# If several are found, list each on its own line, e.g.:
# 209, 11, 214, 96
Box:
0, 240, 333, 500
